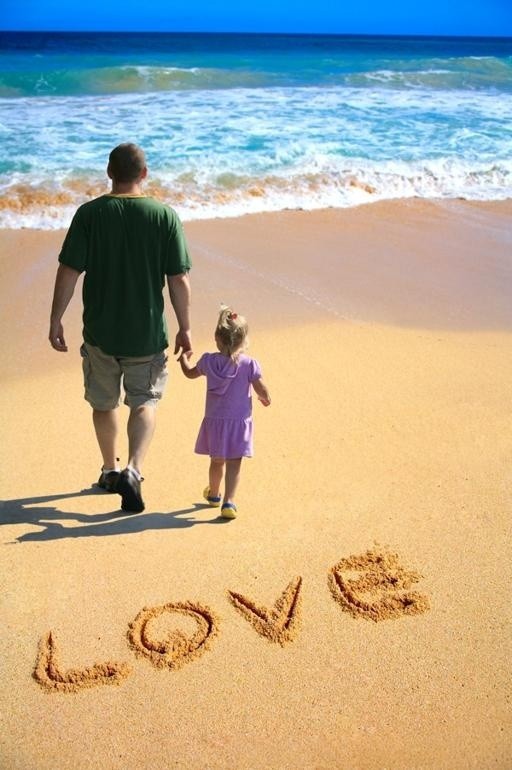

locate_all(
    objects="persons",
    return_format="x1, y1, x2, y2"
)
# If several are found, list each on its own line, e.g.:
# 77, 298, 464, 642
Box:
47, 143, 193, 513
178, 307, 273, 518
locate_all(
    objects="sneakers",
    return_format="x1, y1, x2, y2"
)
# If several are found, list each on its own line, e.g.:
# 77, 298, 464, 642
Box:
203, 486, 221, 507
221, 503, 237, 518
113, 470, 144, 512
98, 471, 118, 493
